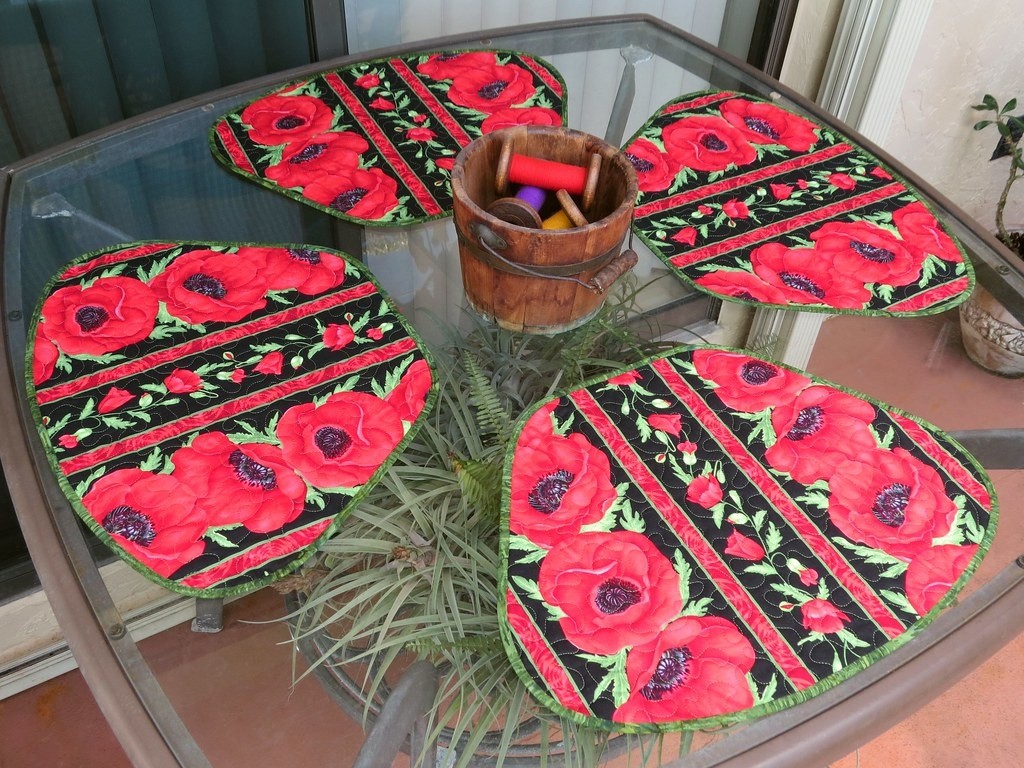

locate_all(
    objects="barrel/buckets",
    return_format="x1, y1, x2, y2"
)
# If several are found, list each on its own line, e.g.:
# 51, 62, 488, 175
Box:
451, 125, 639, 335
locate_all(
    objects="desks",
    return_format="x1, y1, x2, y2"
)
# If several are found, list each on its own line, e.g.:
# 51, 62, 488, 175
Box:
0, 15, 1024, 768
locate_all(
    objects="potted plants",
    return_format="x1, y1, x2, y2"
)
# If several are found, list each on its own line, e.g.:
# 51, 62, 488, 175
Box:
956, 94, 1024, 380
